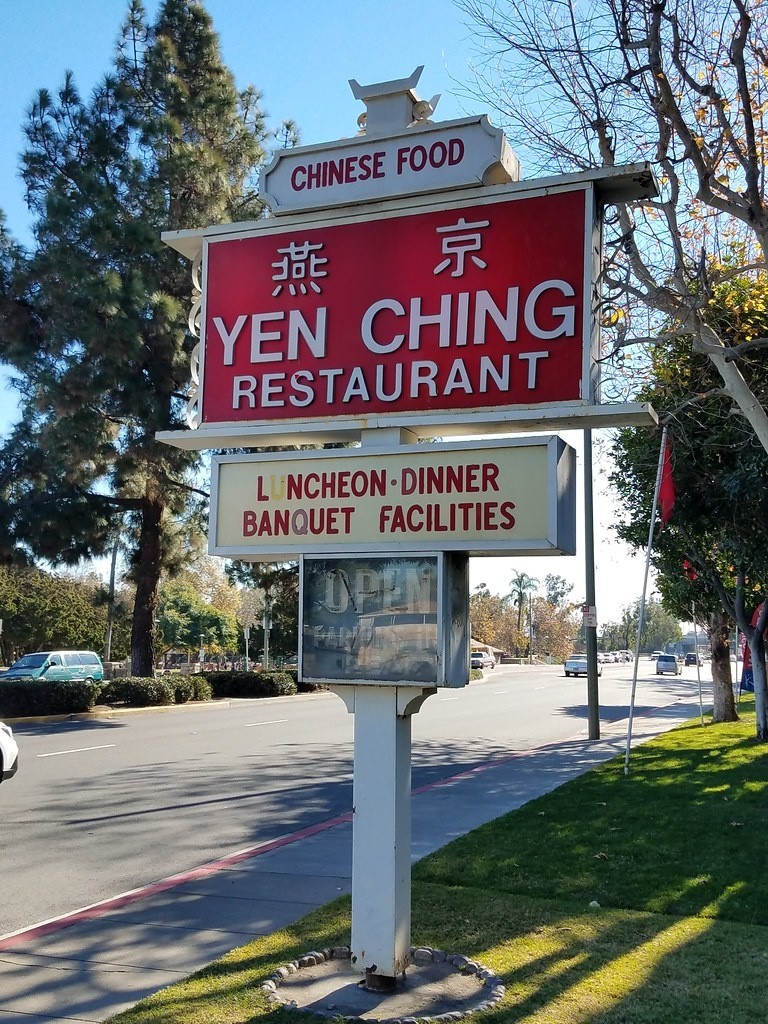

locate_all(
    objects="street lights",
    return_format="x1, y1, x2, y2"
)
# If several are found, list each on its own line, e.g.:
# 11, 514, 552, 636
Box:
199, 634, 206, 672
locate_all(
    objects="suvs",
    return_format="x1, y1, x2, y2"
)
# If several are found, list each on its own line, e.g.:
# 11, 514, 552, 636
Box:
656, 654, 683, 675
684, 652, 700, 666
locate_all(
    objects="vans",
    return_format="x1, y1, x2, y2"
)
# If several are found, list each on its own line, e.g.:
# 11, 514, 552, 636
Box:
0, 651, 105, 691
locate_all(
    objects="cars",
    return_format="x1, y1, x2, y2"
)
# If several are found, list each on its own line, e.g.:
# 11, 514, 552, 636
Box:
609, 651, 623, 663
597, 652, 606, 664
618, 649, 634, 662
470, 652, 496, 670
603, 653, 615, 663
0, 721, 20, 784
701, 650, 744, 663
650, 650, 664, 661
564, 654, 603, 677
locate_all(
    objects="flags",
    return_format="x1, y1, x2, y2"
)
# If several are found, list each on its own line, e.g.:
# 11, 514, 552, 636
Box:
658, 434, 674, 530
